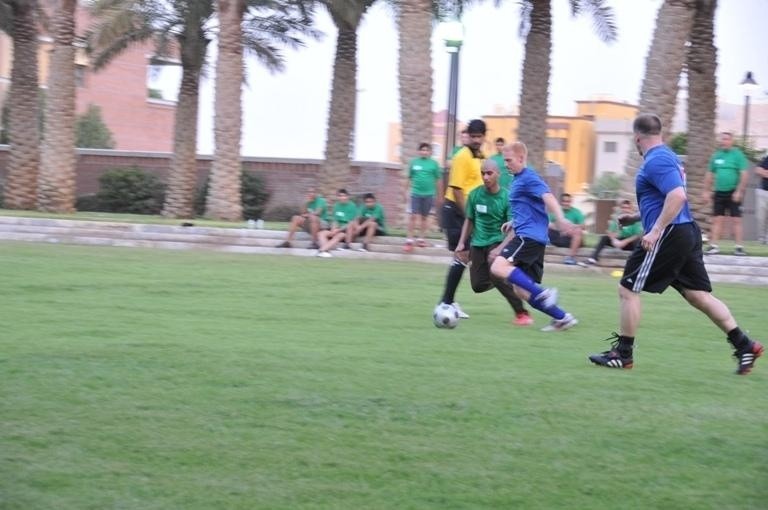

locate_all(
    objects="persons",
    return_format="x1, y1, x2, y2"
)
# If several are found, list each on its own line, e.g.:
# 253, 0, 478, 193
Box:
404, 118, 585, 331
274, 186, 387, 252
590, 115, 763, 376
580, 132, 768, 266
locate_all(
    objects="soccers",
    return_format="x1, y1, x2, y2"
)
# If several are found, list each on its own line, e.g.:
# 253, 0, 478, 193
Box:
433, 304, 457, 329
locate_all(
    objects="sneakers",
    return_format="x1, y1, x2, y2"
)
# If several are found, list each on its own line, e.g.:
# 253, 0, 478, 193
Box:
707, 243, 745, 256
566, 256, 597, 268
589, 350, 633, 370
731, 342, 764, 374
439, 287, 576, 331
276, 238, 426, 257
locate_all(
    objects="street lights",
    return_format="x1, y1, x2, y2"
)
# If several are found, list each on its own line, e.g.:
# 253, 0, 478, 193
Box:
737, 70, 762, 149
439, 20, 466, 234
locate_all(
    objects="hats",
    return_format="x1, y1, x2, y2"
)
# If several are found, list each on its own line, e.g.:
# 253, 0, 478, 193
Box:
460, 119, 485, 134
